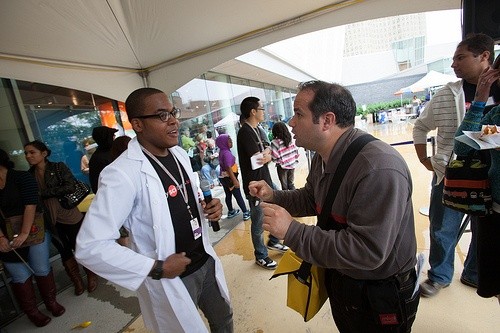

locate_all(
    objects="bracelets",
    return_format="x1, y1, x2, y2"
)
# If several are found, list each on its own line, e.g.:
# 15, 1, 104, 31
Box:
469, 100, 487, 109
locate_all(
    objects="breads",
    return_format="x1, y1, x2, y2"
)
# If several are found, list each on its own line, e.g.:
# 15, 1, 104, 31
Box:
484, 124, 499, 134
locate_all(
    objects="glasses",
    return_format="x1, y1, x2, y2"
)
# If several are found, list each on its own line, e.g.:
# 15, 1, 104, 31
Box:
134, 108, 181, 122
253, 108, 265, 112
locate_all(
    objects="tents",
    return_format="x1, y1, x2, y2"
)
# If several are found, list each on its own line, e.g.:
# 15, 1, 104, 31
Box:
394, 69, 463, 107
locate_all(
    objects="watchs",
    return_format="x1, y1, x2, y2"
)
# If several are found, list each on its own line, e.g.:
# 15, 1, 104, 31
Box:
151, 260, 164, 280
419, 156, 428, 163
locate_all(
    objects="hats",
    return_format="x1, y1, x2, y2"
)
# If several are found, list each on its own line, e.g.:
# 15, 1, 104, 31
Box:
92, 126, 119, 137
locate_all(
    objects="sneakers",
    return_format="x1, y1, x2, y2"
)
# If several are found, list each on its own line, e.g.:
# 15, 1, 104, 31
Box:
242, 209, 251, 220
228, 208, 241, 218
255, 255, 278, 270
266, 240, 290, 252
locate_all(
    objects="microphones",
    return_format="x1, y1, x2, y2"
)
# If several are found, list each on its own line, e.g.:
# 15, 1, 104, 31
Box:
200, 180, 221, 232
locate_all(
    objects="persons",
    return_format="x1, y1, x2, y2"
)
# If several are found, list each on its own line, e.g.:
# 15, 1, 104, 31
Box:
0, 146, 66, 328
74, 87, 233, 333
413, 33, 494, 297
186, 96, 298, 220
81, 125, 132, 248
237, 96, 291, 269
24, 140, 97, 296
246, 80, 420, 333
455, 53, 500, 305
410, 95, 422, 117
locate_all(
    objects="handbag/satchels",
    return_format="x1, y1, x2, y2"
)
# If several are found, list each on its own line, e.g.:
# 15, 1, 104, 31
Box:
441, 149, 492, 212
269, 247, 329, 322
6, 211, 45, 248
55, 162, 91, 210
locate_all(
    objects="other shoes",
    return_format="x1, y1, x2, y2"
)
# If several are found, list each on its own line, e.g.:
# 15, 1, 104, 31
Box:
418, 278, 450, 298
460, 276, 479, 288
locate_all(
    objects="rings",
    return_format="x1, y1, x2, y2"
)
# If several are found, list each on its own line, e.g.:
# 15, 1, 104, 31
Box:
480, 81, 485, 85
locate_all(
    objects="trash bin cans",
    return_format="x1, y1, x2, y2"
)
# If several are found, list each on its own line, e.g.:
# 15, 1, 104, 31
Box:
372, 111, 379, 123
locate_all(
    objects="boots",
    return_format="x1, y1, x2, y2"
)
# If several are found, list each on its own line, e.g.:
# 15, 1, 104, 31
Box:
84, 266, 96, 293
14, 273, 52, 327
33, 267, 65, 317
61, 258, 85, 296
